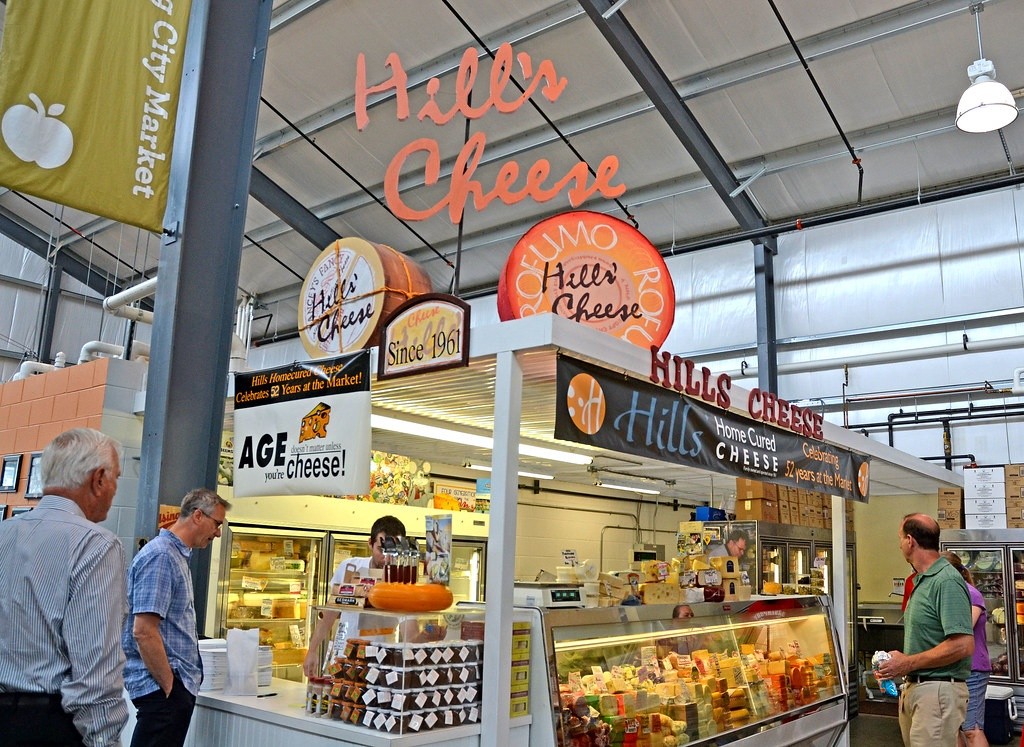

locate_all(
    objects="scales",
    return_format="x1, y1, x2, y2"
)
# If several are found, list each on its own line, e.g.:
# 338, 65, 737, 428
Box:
513, 582, 589, 608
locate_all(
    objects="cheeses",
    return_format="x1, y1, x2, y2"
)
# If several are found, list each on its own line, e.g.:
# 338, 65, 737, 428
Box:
597, 556, 752, 608
555, 645, 840, 747
223, 541, 310, 665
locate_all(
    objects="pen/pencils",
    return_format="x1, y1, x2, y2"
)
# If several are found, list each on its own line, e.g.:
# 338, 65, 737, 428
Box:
257, 693, 276, 698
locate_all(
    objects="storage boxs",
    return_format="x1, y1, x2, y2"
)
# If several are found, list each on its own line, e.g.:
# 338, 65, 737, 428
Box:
937, 462, 1024, 530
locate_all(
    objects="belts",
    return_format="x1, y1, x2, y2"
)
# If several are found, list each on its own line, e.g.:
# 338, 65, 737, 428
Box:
904, 673, 966, 683
0, 695, 50, 706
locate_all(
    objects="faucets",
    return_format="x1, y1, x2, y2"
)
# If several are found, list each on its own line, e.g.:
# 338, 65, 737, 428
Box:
888, 592, 904, 597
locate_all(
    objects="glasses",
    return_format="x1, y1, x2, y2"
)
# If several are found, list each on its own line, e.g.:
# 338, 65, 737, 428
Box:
734, 540, 745, 556
192, 506, 223, 531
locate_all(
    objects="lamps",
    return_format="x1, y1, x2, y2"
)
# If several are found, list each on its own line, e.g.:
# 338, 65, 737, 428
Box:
955, 0, 1019, 133
591, 480, 664, 495
459, 458, 559, 481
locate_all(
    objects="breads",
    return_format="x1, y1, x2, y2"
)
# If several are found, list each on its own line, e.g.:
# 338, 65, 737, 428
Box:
953, 551, 1024, 677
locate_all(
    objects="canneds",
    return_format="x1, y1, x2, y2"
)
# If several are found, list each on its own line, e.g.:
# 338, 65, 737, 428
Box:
330, 639, 484, 734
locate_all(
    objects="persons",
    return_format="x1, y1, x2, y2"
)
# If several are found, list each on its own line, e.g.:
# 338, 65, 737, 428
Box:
673, 605, 694, 618
707, 530, 747, 565
303, 515, 406, 677
0, 427, 130, 747
875, 512, 976, 747
939, 551, 992, 747
121, 487, 231, 747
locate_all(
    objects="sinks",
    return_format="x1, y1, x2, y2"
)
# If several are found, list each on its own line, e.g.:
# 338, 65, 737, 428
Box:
857, 601, 905, 654
